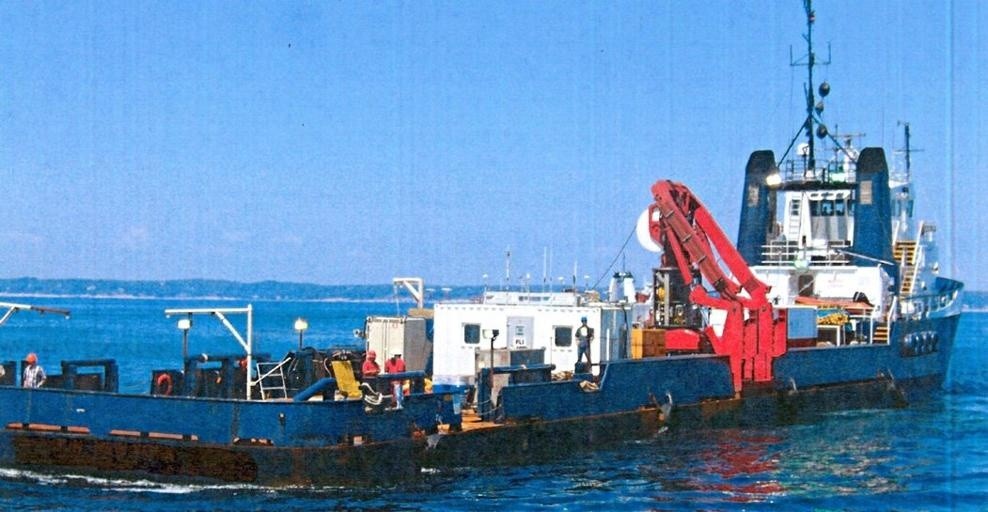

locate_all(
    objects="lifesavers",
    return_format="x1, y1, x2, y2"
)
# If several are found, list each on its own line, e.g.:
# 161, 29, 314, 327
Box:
157, 375, 172, 396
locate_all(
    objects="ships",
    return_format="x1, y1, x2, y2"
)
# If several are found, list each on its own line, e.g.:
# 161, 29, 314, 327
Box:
0, 0, 965, 487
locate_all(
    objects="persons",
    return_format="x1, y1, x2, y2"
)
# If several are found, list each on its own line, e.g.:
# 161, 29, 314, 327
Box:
360, 350, 380, 397
574, 316, 593, 366
384, 351, 407, 408
22, 352, 48, 390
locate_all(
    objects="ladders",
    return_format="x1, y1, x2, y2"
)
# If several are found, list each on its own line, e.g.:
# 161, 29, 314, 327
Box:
256, 363, 287, 400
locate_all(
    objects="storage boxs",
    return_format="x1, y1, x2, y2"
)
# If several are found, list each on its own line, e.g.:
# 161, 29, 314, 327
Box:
630, 328, 666, 358
